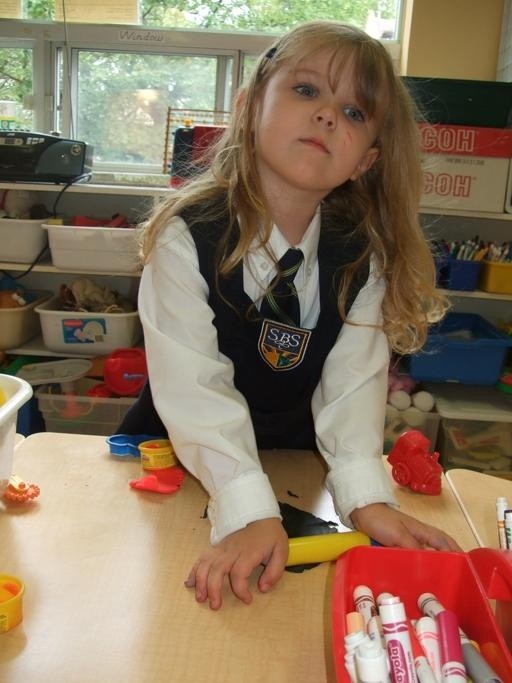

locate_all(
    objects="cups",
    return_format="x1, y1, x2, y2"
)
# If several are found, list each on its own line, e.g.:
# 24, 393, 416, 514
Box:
138, 438, 176, 470
0, 574, 25, 635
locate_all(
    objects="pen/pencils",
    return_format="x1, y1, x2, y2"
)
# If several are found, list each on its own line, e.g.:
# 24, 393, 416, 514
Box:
430, 235, 512, 263
496, 496, 512, 549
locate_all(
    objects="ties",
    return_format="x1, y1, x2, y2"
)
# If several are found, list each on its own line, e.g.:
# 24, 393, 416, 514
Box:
261, 249, 305, 330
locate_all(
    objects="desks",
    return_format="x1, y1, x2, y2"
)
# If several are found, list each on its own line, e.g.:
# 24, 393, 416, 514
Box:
443, 468, 511, 548
2, 425, 483, 681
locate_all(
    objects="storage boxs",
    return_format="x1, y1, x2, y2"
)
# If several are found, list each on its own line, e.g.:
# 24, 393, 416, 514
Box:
404, 309, 507, 387
437, 395, 510, 478
432, 257, 484, 291
484, 259, 511, 292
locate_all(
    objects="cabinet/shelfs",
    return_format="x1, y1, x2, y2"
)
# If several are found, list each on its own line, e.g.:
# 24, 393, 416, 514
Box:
0, 178, 177, 437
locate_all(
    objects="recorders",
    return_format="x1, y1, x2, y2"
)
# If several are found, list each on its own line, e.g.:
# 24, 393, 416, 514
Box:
0, 128, 94, 185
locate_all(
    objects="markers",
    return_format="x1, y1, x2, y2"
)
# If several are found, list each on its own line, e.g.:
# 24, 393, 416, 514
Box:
344, 584, 503, 683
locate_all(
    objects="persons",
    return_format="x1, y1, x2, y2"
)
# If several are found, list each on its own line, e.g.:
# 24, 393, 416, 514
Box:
109, 19, 467, 611
